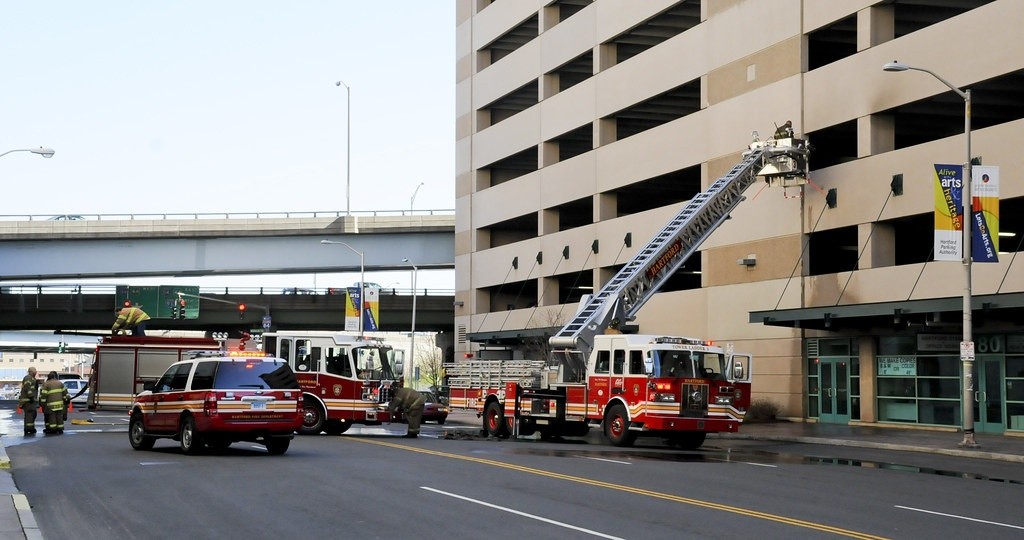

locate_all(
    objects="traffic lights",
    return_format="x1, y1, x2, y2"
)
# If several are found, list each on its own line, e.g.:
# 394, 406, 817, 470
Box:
179, 305, 185, 320
170, 307, 176, 320
239, 304, 245, 320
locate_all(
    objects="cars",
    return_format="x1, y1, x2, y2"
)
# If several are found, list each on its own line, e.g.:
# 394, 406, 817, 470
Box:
48, 214, 85, 221
416, 390, 450, 425
56, 372, 91, 404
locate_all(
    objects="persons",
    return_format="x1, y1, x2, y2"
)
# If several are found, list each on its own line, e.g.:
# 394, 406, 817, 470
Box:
112, 307, 151, 336
673, 354, 701, 378
774, 121, 794, 140
384, 387, 427, 438
40, 371, 70, 434
20, 367, 38, 433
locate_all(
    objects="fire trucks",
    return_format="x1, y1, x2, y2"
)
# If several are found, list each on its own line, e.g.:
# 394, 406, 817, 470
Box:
76, 331, 406, 435
476, 121, 813, 451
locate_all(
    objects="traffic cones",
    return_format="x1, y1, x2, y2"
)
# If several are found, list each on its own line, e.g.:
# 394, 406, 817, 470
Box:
68, 400, 74, 413
16, 406, 23, 414
38, 406, 43, 413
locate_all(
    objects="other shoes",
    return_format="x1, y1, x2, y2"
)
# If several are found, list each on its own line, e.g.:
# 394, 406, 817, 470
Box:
24, 428, 36, 435
402, 433, 417, 438
43, 428, 64, 435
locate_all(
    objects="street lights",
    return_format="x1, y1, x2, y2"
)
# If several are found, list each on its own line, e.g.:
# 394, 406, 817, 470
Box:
401, 258, 417, 388
320, 240, 365, 340
334, 80, 350, 215
410, 181, 425, 293
881, 63, 980, 448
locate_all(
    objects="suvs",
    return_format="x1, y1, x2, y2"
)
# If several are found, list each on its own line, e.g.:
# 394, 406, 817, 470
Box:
126, 349, 306, 455
